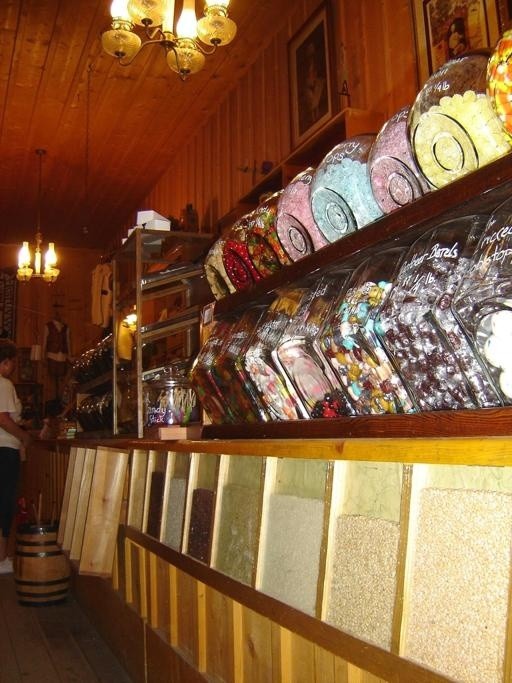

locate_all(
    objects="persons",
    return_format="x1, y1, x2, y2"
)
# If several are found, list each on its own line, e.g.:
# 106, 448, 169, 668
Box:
43, 299, 72, 400
0, 337, 32, 573
302, 43, 322, 121
449, 24, 463, 49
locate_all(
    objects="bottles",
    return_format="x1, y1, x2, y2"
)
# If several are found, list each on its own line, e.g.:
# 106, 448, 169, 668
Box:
149, 364, 200, 426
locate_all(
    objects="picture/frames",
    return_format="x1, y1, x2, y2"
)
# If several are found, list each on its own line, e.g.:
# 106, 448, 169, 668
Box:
286, 0, 339, 152
408, 0, 505, 97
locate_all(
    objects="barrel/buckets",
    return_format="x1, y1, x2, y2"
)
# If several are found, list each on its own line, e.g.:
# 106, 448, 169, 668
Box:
15, 521, 73, 606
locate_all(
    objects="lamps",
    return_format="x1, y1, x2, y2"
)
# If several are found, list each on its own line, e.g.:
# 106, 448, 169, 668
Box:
17, 149, 60, 288
100, 0, 237, 82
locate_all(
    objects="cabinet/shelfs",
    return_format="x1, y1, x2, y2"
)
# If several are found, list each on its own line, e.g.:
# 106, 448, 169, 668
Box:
335, 0, 418, 138
202, 106, 512, 440
108, 230, 216, 440
72, 368, 113, 434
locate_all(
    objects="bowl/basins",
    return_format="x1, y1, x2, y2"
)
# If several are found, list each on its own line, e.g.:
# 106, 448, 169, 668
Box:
365, 104, 433, 214
243, 190, 291, 278
223, 212, 260, 289
272, 166, 327, 263
410, 51, 512, 194
202, 226, 238, 298
310, 135, 383, 243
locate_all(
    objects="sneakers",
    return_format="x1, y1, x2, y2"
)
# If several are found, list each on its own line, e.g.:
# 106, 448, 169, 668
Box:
0, 557, 14, 574
